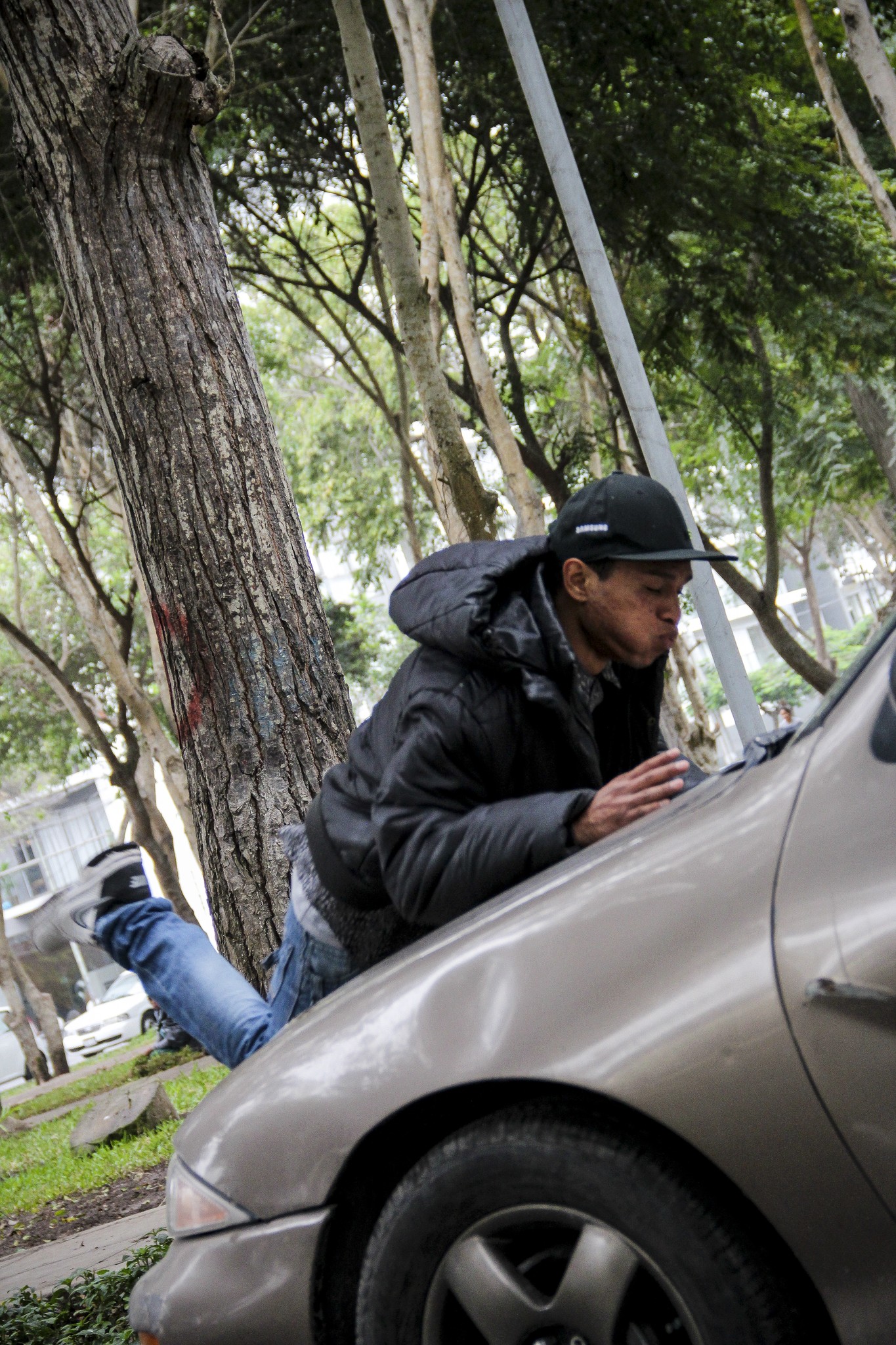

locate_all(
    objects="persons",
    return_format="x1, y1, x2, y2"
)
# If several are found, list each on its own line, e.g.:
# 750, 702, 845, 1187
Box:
777, 707, 804, 728
141, 995, 189, 1057
31, 468, 737, 1072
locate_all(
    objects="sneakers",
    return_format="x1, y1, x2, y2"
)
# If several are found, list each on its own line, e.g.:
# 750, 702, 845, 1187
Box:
32, 841, 152, 957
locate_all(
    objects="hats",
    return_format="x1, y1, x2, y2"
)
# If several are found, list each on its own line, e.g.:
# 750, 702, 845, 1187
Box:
548, 471, 738, 564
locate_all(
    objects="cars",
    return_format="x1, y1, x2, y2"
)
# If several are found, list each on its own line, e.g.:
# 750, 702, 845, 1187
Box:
64, 968, 158, 1058
124, 602, 896, 1345
